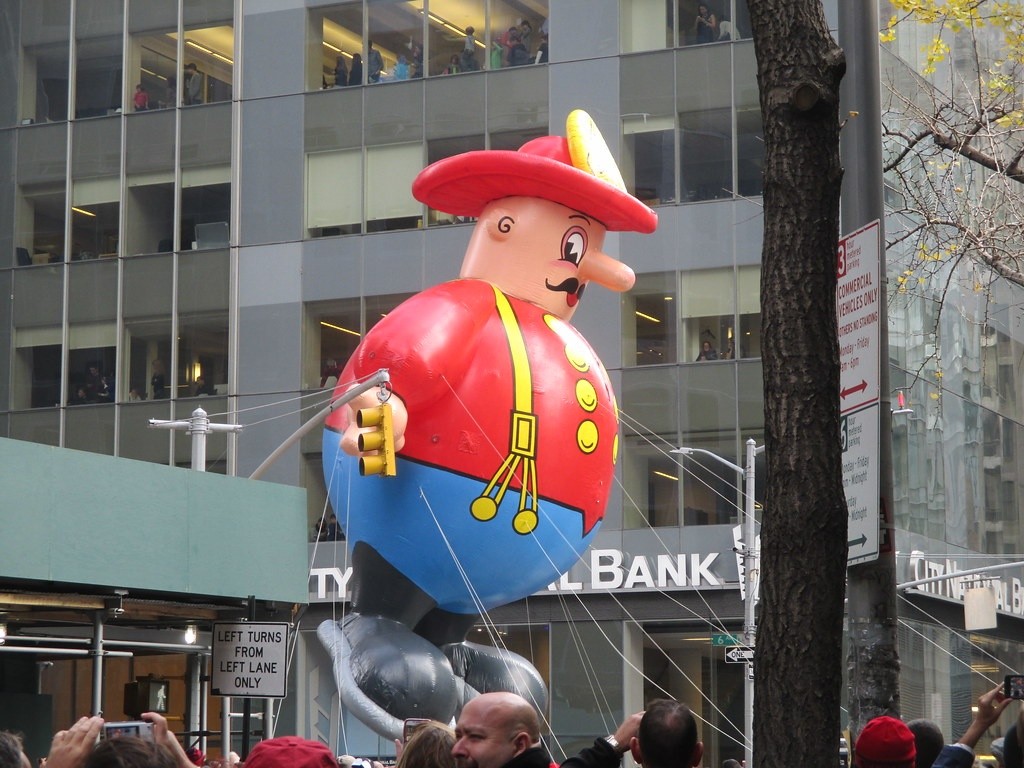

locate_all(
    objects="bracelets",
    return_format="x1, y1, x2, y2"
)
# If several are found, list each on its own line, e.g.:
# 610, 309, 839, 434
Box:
607, 735, 622, 752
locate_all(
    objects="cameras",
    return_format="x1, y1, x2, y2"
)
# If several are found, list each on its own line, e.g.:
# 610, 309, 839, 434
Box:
104, 721, 155, 744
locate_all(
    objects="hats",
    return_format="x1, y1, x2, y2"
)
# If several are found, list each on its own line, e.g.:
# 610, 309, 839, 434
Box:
243, 737, 337, 768
853, 715, 916, 768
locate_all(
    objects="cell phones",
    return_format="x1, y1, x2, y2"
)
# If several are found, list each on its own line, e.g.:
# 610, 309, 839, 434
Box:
1005, 675, 1024, 700
403, 718, 431, 748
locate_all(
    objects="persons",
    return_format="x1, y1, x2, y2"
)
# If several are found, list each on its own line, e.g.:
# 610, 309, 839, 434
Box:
695, 4, 740, 43
76, 365, 114, 403
311, 514, 344, 542
130, 389, 141, 401
134, 84, 149, 111
46, 712, 197, 768
696, 336, 746, 360
0, 681, 1024, 768
334, 21, 549, 86
196, 376, 215, 396
321, 358, 340, 385
152, 360, 166, 399
167, 63, 200, 108
